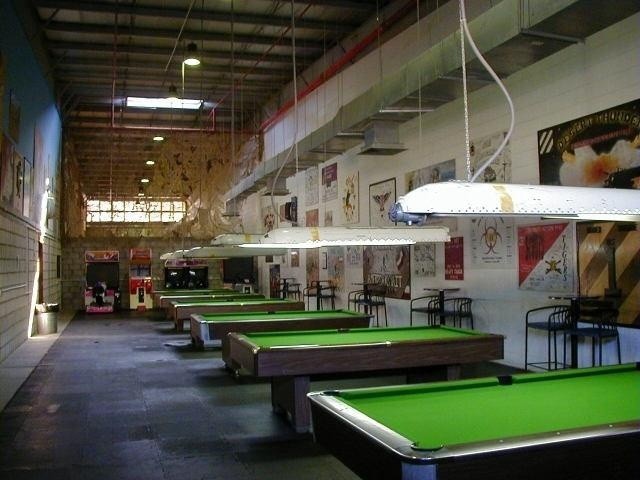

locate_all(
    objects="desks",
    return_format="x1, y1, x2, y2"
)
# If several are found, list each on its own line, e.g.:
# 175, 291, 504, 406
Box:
306, 361, 639, 477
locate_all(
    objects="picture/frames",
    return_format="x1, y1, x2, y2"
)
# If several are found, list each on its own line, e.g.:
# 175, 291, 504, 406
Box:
574, 219, 639, 331
